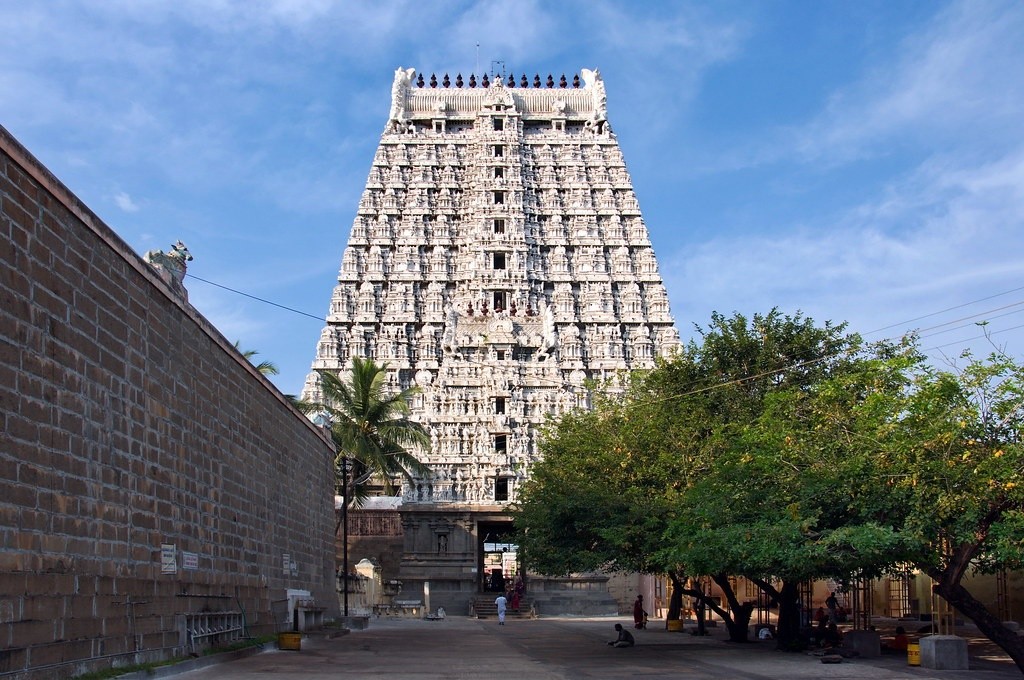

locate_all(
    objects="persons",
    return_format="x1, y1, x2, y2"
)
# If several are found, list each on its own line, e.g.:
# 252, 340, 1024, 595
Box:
633, 595, 649, 630
808, 592, 848, 652
482, 573, 524, 613
438, 537, 447, 557
754, 624, 774, 640
692, 581, 705, 635
495, 592, 507, 625
607, 623, 635, 648
864, 625, 911, 656
301, 68, 685, 500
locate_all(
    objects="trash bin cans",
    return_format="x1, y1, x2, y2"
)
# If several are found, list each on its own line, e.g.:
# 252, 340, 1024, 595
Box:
906, 636, 921, 666
